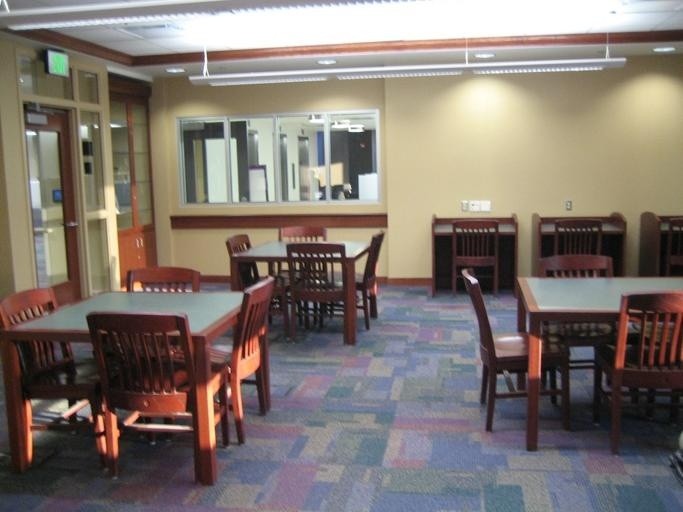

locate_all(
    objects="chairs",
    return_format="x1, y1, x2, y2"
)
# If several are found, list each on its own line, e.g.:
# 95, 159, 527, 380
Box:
425, 209, 683, 296
225, 224, 384, 345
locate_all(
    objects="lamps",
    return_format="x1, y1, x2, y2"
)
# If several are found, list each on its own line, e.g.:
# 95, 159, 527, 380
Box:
308, 115, 364, 134
